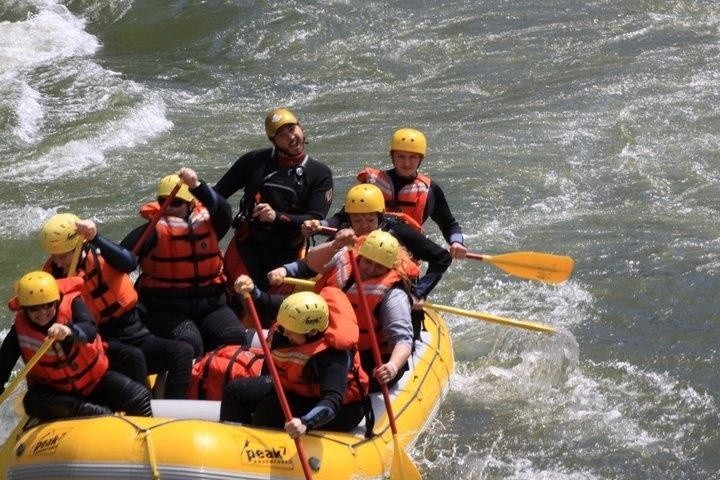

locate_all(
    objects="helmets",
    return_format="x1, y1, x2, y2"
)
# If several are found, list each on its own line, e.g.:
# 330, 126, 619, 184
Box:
276, 290, 330, 335
263, 106, 299, 140
39, 212, 83, 256
13, 270, 62, 308
389, 127, 429, 156
155, 173, 196, 200
357, 229, 401, 270
343, 182, 386, 215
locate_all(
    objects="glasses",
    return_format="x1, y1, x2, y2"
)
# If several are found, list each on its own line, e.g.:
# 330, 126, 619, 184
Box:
157, 195, 185, 208
26, 302, 57, 313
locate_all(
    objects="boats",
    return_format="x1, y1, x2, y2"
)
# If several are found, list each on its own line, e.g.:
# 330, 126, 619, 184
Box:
0, 301, 461, 480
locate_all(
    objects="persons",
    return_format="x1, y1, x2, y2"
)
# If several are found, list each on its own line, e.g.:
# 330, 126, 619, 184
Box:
1, 108, 466, 441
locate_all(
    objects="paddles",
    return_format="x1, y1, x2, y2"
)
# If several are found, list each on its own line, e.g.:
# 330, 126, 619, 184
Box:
283, 275, 559, 334
316, 225, 574, 284
347, 245, 423, 479
